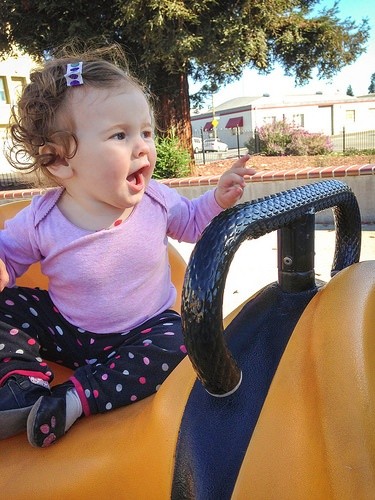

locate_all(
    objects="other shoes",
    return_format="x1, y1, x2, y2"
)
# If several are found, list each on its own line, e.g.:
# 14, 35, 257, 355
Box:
27, 380, 84, 449
0, 374, 51, 440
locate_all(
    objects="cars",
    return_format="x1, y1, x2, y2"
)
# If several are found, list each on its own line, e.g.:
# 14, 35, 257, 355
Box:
192, 137, 203, 154
203, 138, 228, 154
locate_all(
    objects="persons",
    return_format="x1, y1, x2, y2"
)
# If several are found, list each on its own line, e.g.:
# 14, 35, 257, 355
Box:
0, 42, 257, 449
